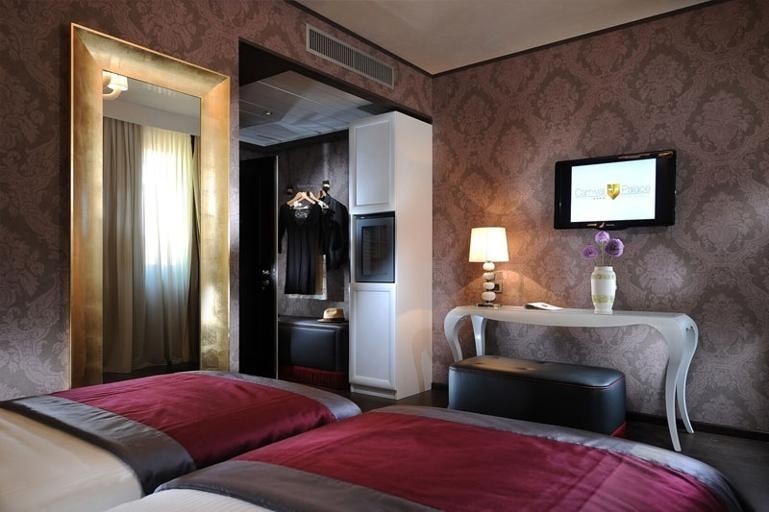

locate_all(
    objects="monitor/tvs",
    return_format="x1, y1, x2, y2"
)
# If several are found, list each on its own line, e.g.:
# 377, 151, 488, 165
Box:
552, 150, 676, 231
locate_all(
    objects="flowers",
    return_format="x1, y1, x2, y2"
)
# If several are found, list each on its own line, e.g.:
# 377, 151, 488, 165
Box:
583, 230, 625, 266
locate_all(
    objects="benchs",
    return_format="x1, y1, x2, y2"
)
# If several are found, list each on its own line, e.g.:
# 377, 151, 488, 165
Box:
448, 354, 627, 440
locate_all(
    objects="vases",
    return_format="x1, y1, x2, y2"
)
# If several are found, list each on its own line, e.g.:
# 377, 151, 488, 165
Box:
591, 266, 617, 314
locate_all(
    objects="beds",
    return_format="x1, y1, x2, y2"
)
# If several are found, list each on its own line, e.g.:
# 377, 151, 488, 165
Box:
2, 367, 361, 512
109, 406, 751, 510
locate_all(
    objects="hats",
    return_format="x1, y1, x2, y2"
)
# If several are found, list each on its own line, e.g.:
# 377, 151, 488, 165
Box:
316, 308, 349, 323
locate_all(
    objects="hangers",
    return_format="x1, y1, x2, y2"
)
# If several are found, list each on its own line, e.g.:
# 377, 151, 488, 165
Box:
279, 185, 342, 219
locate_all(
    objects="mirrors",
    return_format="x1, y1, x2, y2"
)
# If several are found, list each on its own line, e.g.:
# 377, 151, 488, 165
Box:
70, 21, 232, 391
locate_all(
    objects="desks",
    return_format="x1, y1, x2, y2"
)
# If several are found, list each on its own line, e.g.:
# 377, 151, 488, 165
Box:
443, 303, 699, 452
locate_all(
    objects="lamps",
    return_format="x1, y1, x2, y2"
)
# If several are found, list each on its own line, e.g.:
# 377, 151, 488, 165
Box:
468, 227, 509, 309
